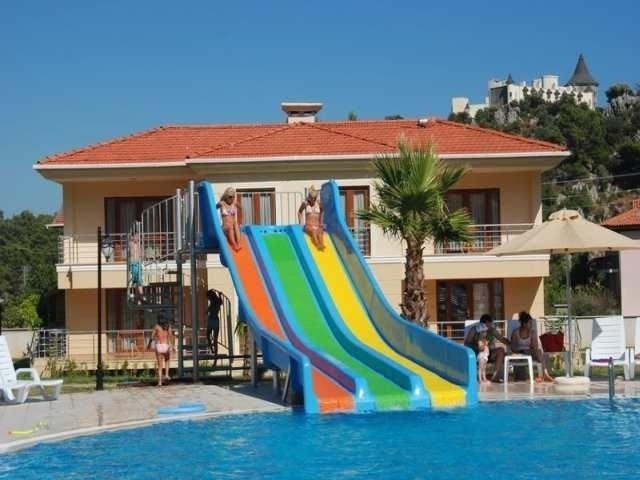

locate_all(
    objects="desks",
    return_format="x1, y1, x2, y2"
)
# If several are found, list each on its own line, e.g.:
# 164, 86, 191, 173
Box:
543, 350, 570, 380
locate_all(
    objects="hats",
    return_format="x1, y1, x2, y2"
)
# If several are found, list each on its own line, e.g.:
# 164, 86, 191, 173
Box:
476, 322, 488, 332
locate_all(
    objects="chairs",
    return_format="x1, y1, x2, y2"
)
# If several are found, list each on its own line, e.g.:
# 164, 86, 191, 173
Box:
584, 315, 640, 381
463, 318, 543, 384
0, 335, 64, 405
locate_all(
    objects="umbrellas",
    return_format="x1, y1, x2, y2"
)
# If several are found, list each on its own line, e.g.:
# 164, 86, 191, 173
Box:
481, 206, 640, 379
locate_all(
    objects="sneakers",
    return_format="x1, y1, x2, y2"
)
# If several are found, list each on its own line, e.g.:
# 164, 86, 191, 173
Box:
491, 378, 503, 382
486, 381, 490, 384
535, 378, 542, 383
480, 382, 484, 384
544, 377, 554, 382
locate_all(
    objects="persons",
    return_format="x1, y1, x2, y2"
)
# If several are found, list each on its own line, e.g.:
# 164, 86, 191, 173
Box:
126, 231, 149, 302
465, 313, 510, 384
203, 289, 223, 366
214, 187, 246, 251
147, 315, 172, 386
296, 187, 327, 252
510, 312, 554, 382
475, 328, 491, 383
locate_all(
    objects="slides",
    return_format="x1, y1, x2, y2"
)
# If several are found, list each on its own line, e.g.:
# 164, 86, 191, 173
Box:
196, 180, 371, 415
288, 179, 478, 414
245, 223, 431, 414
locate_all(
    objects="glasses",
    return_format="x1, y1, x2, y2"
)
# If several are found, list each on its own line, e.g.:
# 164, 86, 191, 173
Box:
229, 196, 235, 198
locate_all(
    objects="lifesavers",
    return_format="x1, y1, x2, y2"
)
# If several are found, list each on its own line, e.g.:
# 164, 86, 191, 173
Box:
158, 402, 206, 414
